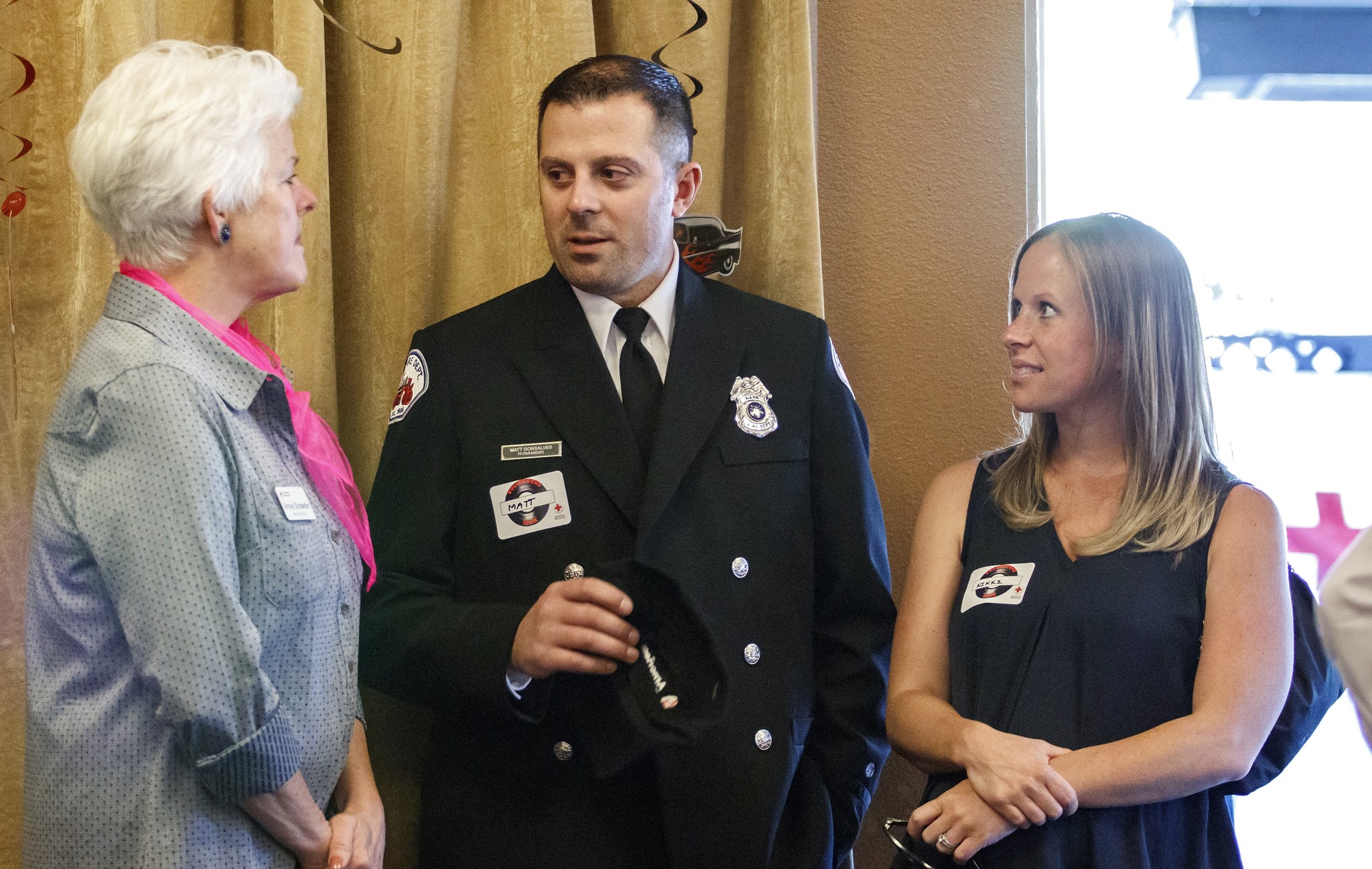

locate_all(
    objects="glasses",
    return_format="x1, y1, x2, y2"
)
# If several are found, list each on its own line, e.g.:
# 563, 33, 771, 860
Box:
882, 817, 979, 869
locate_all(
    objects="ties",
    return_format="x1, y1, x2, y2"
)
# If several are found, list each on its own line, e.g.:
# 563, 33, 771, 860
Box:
612, 307, 663, 474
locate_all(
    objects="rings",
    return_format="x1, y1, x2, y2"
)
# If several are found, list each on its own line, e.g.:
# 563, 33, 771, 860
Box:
938, 833, 957, 849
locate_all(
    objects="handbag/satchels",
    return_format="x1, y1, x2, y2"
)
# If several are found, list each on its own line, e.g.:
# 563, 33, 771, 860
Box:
1195, 479, 1346, 796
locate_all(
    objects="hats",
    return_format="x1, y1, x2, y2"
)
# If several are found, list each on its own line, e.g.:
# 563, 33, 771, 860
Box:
578, 560, 732, 776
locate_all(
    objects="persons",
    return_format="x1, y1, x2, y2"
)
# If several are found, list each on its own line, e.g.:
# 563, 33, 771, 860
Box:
885, 213, 1294, 869
21, 36, 387, 869
1318, 523, 1372, 753
356, 55, 899, 869
